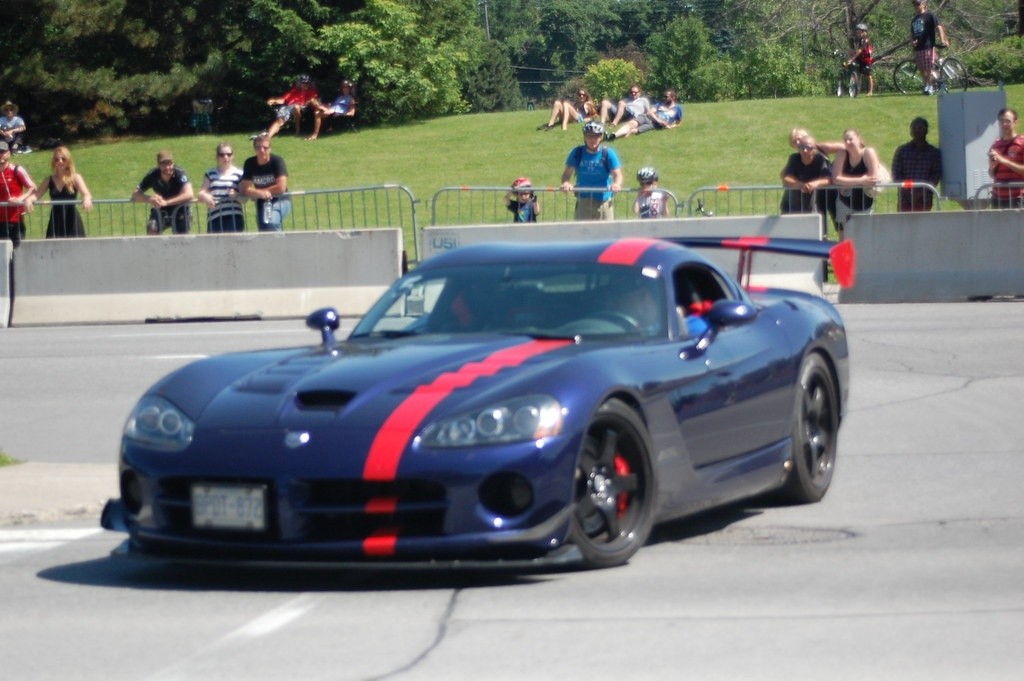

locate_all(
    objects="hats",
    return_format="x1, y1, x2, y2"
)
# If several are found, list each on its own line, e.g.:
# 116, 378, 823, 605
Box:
1, 101, 19, 115
301, 74, 311, 82
0, 141, 9, 151
157, 150, 172, 163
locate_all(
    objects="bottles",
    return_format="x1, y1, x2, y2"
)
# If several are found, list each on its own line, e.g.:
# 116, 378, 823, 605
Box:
263, 199, 273, 224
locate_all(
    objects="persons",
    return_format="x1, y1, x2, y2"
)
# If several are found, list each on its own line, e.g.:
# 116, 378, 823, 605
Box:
631, 167, 670, 218
505, 177, 540, 222
131, 151, 194, 235
986, 108, 1024, 300
847, 22, 873, 97
604, 89, 683, 141
260, 73, 318, 138
545, 88, 597, 132
23, 144, 92, 238
243, 135, 292, 232
910, 0, 946, 95
0, 100, 26, 154
615, 276, 707, 336
777, 126, 879, 241
561, 120, 623, 220
599, 84, 650, 128
304, 79, 355, 140
0, 141, 36, 247
891, 116, 942, 212
197, 143, 249, 233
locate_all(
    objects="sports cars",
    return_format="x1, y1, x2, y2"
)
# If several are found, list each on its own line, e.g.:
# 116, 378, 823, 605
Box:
99, 232, 857, 573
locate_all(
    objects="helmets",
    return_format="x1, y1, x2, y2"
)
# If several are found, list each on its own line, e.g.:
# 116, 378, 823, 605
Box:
855, 24, 867, 30
913, 0, 927, 5
584, 122, 604, 136
512, 179, 531, 190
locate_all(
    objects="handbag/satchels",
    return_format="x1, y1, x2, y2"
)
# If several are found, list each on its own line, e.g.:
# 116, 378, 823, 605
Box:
860, 149, 890, 197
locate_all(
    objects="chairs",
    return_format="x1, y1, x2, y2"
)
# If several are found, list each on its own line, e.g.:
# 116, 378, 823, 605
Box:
270, 84, 358, 134
483, 286, 556, 331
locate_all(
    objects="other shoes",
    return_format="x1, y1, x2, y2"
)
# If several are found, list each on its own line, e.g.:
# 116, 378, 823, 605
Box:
11, 148, 17, 155
924, 86, 933, 97
607, 133, 616, 141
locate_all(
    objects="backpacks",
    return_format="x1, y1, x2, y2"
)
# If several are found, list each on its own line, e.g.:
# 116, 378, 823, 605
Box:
40, 137, 57, 150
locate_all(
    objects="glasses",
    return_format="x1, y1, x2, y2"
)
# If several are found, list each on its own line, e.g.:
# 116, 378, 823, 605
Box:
55, 157, 66, 163
630, 90, 640, 94
4, 108, 13, 111
579, 92, 586, 96
799, 145, 815, 152
218, 152, 232, 157
914, 4, 920, 6
638, 167, 658, 181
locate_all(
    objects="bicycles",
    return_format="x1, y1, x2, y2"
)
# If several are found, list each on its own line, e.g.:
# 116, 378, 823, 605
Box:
832, 49, 860, 98
893, 42, 968, 96
